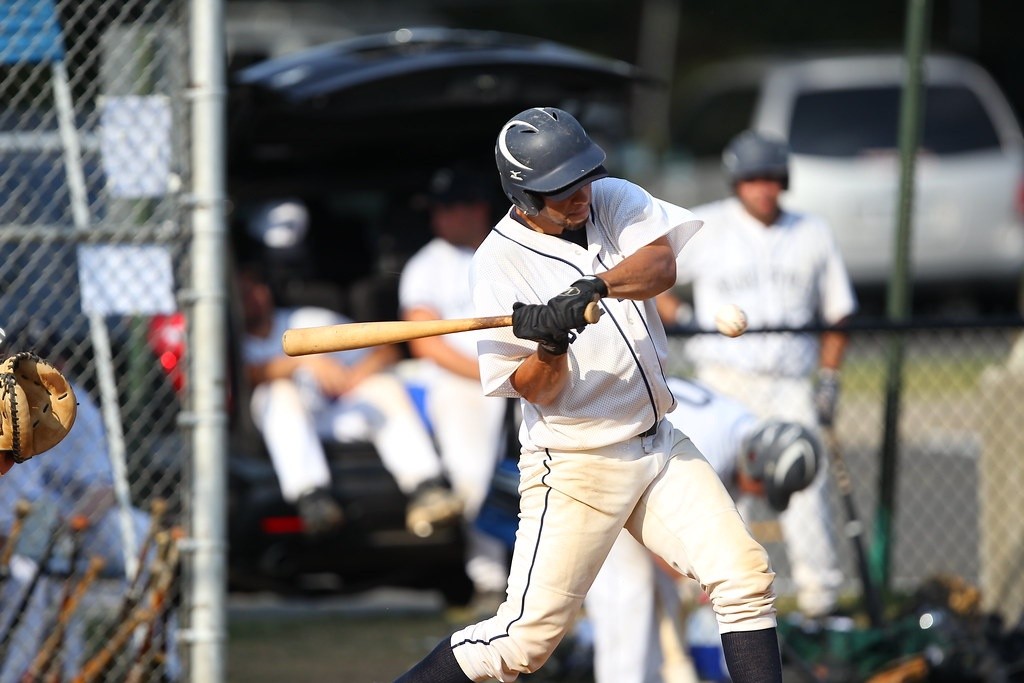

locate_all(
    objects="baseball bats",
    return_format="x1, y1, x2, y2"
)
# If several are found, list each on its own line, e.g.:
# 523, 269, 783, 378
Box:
0, 495, 187, 682
282, 300, 601, 357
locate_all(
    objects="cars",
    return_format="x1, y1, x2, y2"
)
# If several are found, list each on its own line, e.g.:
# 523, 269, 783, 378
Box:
126, 25, 664, 608
661, 48, 1024, 332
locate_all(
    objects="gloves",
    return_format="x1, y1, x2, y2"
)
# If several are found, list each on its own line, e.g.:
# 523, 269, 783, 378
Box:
512, 302, 570, 357
548, 273, 608, 331
815, 365, 842, 431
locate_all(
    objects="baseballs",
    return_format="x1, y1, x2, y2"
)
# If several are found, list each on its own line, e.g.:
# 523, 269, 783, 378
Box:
715, 303, 747, 338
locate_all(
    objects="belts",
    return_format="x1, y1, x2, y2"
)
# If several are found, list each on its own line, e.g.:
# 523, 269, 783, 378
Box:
636, 421, 659, 438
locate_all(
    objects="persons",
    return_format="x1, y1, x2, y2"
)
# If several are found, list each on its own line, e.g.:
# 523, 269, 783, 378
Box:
0, 375, 181, 683
397, 151, 533, 589
237, 278, 465, 540
394, 107, 785, 683
656, 126, 861, 617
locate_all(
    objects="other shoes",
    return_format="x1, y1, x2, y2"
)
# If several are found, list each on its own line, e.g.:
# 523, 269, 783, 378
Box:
405, 477, 463, 537
297, 483, 341, 534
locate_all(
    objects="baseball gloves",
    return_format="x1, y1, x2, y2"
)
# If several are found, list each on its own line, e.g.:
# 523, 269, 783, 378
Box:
0, 347, 78, 464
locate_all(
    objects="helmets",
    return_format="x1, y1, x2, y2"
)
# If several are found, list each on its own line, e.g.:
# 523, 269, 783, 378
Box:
746, 423, 820, 513
495, 106, 610, 216
719, 130, 790, 195
